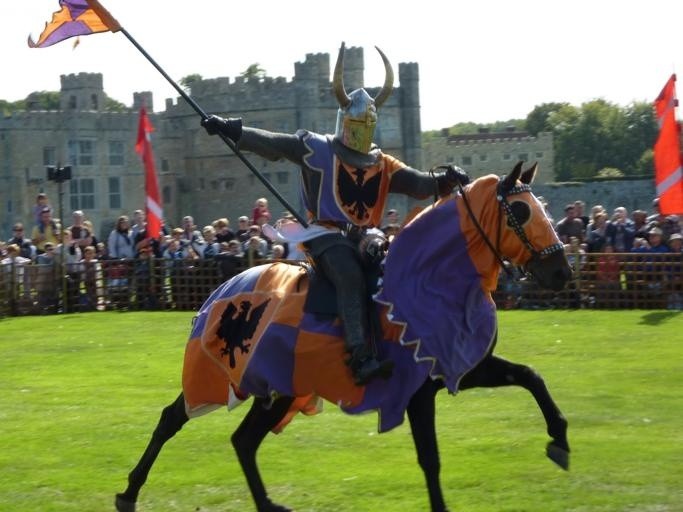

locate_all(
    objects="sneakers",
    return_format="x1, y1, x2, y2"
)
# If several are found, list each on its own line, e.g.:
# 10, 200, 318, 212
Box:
352, 360, 388, 383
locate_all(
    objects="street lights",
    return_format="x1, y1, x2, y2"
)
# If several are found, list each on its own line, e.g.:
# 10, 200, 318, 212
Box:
47, 165, 72, 313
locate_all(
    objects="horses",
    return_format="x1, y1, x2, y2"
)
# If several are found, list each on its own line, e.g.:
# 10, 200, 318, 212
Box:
115, 161, 574, 512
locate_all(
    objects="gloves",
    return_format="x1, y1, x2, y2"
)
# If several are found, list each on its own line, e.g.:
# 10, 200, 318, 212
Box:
201, 114, 242, 140
439, 166, 469, 192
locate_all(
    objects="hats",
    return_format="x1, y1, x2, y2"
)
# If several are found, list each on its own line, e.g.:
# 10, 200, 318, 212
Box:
648, 227, 663, 234
669, 233, 683, 240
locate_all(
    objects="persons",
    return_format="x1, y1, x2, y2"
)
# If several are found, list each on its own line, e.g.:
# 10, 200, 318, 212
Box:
0, 192, 304, 318
382, 210, 401, 239
198, 37, 468, 383
500, 195, 683, 308
